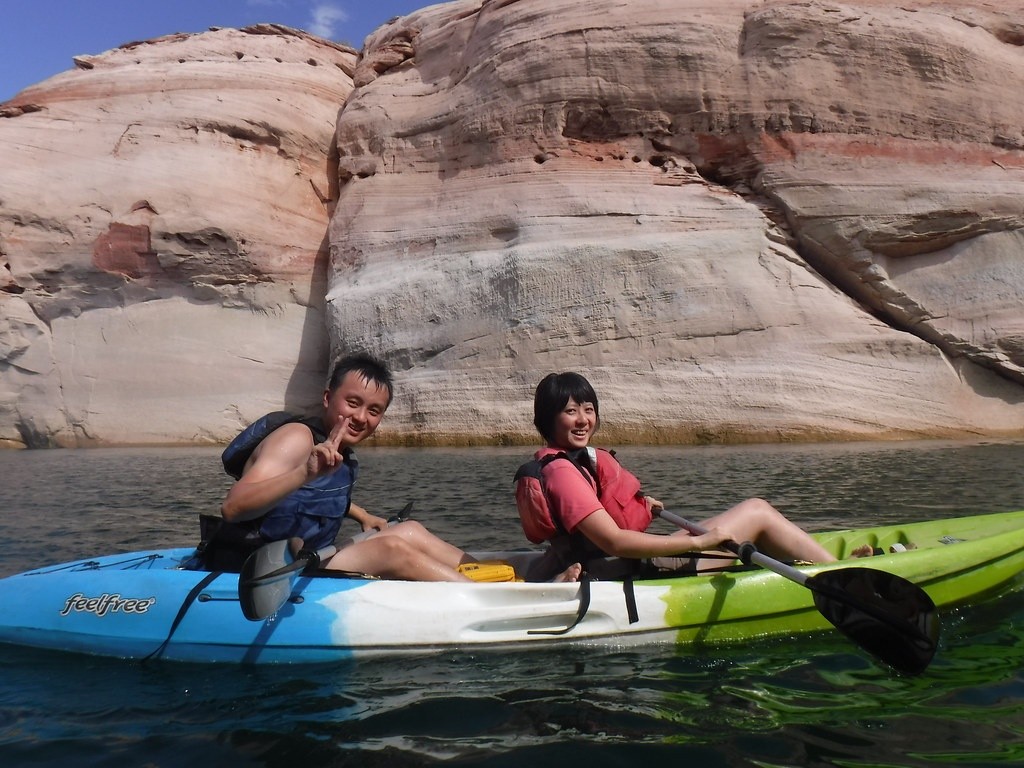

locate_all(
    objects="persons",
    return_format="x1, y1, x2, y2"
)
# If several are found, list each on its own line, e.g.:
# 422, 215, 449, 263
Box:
513, 371, 873, 581
221, 352, 582, 583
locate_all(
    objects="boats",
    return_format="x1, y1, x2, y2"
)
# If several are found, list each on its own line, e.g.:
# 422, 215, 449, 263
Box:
0, 514, 1023, 670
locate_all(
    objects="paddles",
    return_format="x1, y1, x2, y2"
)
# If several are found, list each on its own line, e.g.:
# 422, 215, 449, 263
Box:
598, 447, 944, 678
236, 503, 416, 620
